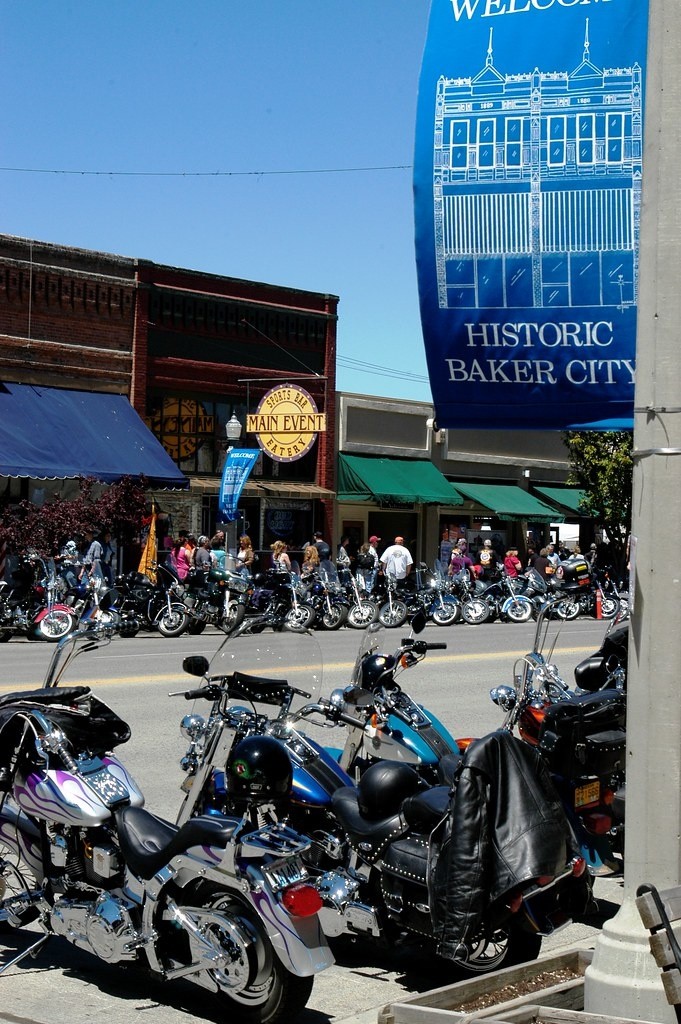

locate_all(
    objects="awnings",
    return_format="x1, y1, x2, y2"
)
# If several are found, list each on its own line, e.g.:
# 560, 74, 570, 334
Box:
446, 482, 566, 526
533, 485, 629, 520
0, 382, 192, 493
336, 451, 464, 507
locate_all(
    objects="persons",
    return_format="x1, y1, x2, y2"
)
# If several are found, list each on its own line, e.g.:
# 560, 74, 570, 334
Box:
0, 526, 628, 581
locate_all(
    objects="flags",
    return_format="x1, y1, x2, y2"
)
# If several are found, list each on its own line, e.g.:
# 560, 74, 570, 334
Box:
137, 498, 159, 585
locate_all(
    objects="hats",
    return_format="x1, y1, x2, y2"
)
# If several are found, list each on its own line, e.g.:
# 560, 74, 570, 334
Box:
369, 536, 381, 541
314, 532, 323, 536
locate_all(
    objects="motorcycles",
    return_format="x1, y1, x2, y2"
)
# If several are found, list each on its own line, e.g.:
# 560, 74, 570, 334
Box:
0, 537, 626, 1022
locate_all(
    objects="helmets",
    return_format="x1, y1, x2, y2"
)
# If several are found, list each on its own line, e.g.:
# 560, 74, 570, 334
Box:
226, 736, 293, 807
357, 761, 431, 820
